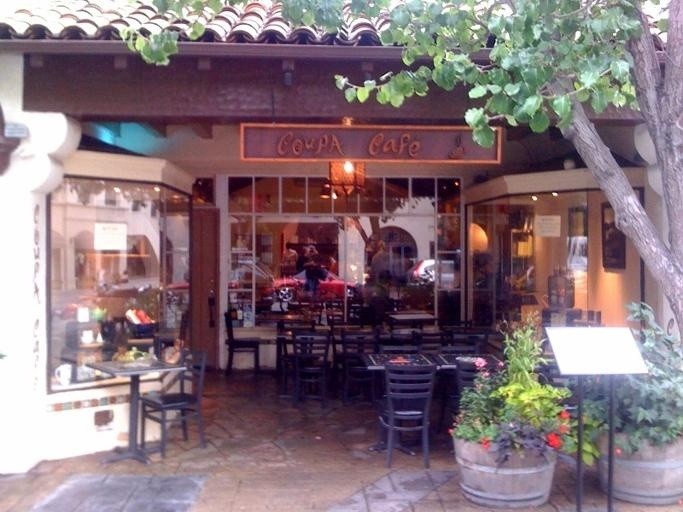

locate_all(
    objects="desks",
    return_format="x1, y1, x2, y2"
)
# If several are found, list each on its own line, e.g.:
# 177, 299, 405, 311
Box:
85, 359, 188, 464
361, 353, 442, 456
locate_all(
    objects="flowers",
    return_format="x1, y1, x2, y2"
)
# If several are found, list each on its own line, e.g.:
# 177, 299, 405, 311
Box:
452, 311, 572, 456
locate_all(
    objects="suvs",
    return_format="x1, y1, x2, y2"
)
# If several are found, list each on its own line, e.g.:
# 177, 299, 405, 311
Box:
162, 262, 273, 313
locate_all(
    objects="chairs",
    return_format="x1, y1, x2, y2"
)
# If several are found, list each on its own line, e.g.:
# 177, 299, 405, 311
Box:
138, 347, 208, 460
380, 363, 437, 467
225, 314, 261, 377
275, 313, 509, 425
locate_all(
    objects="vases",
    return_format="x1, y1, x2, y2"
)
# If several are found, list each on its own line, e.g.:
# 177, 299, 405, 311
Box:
451, 428, 558, 508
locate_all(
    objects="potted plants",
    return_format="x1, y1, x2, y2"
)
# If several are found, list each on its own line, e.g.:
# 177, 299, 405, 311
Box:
595, 301, 683, 506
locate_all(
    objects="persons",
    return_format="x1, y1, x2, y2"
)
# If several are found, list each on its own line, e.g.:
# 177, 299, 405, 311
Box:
281, 243, 322, 293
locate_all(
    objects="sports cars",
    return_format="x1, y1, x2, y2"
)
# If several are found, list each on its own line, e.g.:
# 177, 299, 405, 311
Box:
275, 268, 357, 300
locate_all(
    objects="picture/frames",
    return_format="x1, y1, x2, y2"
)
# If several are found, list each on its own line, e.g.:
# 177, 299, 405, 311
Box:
600, 201, 626, 269
567, 206, 589, 257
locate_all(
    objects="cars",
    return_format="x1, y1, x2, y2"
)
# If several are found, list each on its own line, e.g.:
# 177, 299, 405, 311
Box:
408, 259, 454, 287
